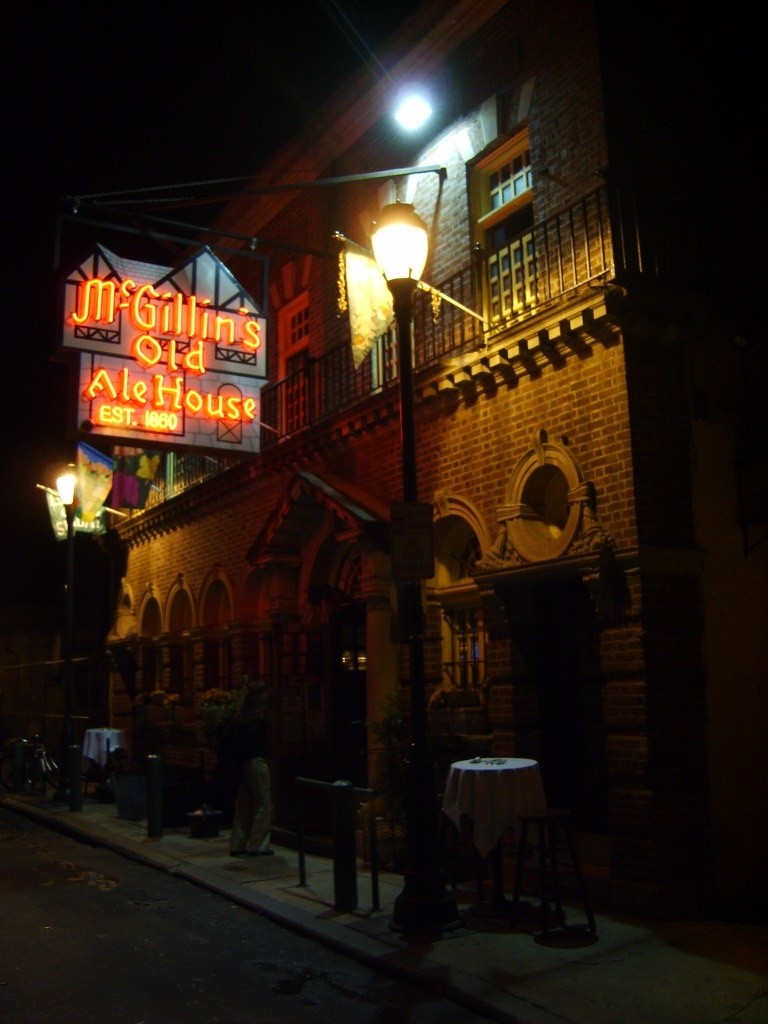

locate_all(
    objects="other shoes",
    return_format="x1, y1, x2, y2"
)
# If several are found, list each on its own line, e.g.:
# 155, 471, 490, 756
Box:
245, 849, 274, 856
230, 849, 246, 856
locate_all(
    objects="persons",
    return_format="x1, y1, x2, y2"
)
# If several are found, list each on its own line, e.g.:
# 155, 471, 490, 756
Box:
228, 683, 280, 856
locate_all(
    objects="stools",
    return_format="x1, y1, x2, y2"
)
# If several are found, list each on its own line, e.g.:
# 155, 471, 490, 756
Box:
511, 810, 596, 941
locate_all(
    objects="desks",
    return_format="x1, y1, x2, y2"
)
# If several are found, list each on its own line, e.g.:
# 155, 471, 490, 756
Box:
82, 727, 129, 800
441, 757, 548, 924
